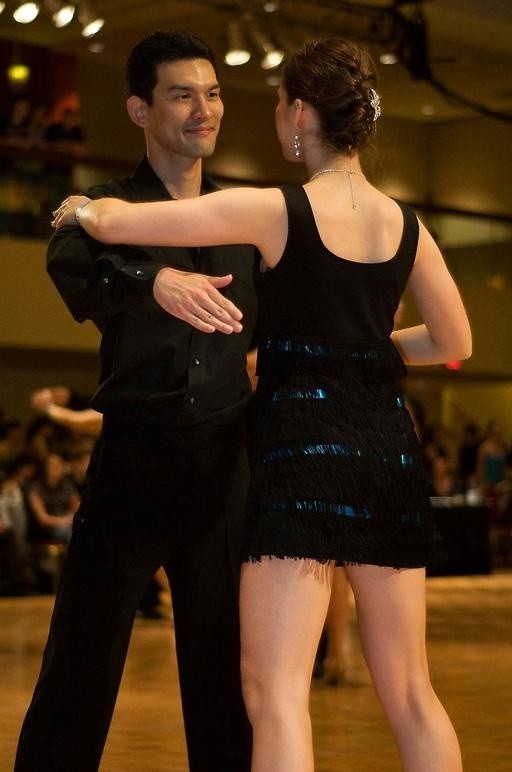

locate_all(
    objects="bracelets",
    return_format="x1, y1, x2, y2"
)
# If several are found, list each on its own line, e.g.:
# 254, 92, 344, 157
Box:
74, 201, 92, 218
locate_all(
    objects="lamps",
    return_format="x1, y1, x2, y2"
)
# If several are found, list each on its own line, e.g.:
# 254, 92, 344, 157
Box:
219, 5, 290, 70
45, 1, 108, 39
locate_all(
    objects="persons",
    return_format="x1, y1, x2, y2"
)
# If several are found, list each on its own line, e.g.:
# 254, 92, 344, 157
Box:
1, 383, 172, 619
12, 32, 259, 771
311, 390, 512, 684
50, 33, 473, 772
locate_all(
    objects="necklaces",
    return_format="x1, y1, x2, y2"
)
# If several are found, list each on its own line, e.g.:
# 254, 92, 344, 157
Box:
309, 166, 366, 213
